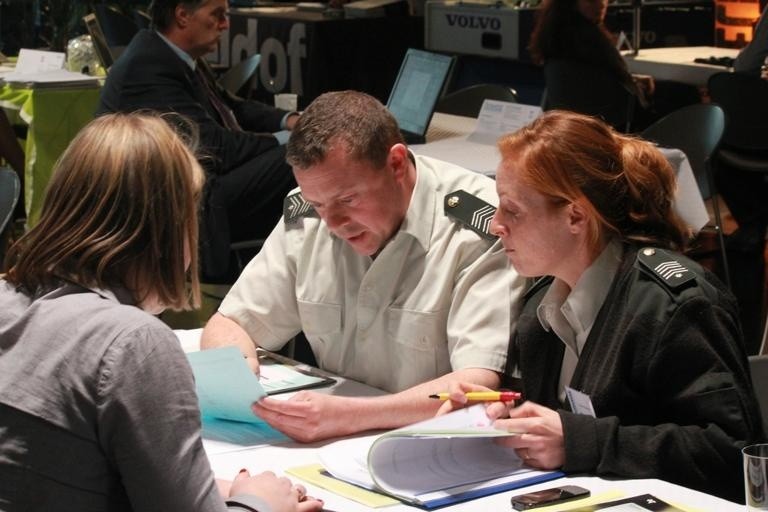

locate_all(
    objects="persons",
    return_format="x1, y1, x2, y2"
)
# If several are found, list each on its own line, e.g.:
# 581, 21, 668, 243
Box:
521, 0, 725, 199
99, 0, 314, 271
0, 106, 323, 511
434, 109, 766, 504
731, 3, 768, 75
195, 88, 526, 444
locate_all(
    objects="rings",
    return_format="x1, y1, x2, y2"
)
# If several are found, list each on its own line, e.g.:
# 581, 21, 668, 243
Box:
523, 448, 530, 460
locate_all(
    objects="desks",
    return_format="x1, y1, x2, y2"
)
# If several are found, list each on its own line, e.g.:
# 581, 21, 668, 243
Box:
0, 55, 107, 235
230, 4, 386, 99
616, 45, 768, 101
169, 326, 768, 512
267, 104, 692, 237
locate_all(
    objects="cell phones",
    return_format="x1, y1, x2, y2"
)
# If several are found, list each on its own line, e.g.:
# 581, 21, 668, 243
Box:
511, 484, 591, 511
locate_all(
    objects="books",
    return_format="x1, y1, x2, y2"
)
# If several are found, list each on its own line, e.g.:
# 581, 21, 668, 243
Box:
322, 396, 540, 511
224, 344, 347, 392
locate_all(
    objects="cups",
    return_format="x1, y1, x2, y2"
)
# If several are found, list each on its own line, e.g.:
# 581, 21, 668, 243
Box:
741, 443, 768, 512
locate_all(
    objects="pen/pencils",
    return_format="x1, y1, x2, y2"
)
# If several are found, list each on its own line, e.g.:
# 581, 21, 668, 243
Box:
428, 391, 521, 400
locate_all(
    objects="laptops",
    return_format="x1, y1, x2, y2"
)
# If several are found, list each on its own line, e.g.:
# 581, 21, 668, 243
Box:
82, 12, 116, 74
383, 46, 456, 146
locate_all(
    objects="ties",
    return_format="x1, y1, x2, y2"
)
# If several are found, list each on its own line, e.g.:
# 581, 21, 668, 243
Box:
196, 65, 240, 131
